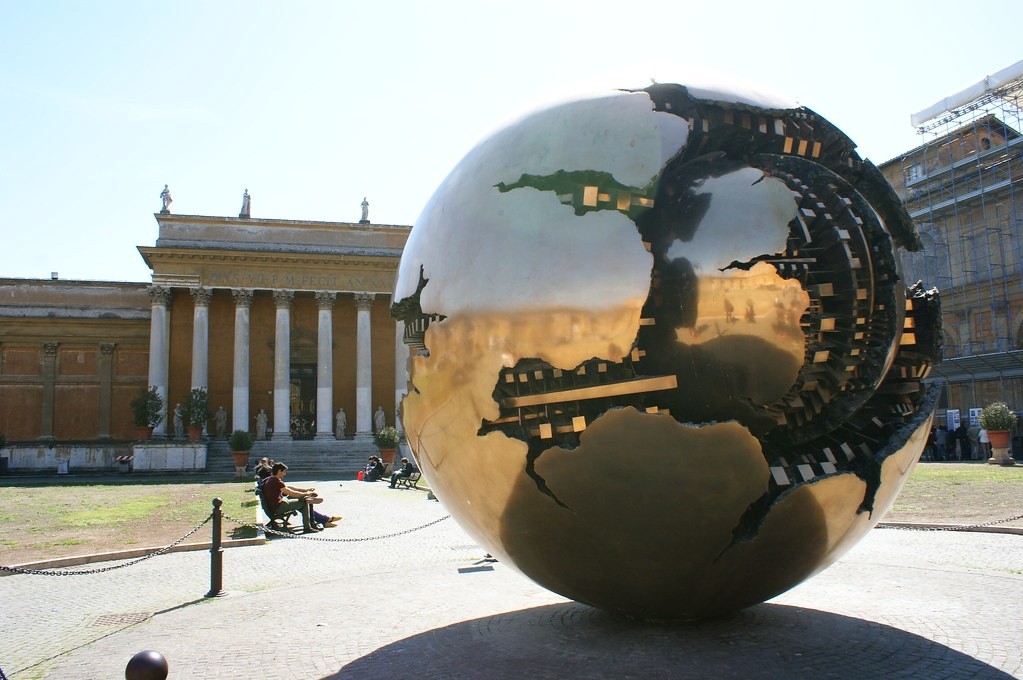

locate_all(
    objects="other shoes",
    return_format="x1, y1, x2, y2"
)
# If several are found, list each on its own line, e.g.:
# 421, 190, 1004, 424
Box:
328, 516, 342, 522
324, 522, 337, 528
388, 485, 395, 489
305, 498, 323, 504
312, 525, 324, 531
303, 528, 319, 533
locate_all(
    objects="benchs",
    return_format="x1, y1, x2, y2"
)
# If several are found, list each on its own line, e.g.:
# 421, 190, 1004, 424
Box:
255, 485, 298, 534
254, 468, 265, 486
397, 464, 422, 490
372, 463, 388, 481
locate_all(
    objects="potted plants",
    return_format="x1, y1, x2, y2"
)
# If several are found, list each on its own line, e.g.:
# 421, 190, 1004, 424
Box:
372, 425, 400, 462
976, 399, 1018, 448
129, 385, 167, 439
229, 431, 255, 466
175, 385, 210, 441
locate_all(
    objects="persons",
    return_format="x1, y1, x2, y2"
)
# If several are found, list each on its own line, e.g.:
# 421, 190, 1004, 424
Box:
364, 455, 385, 481
925, 424, 992, 461
241, 189, 250, 214
374, 406, 385, 432
160, 184, 172, 208
254, 457, 342, 533
254, 409, 266, 437
173, 403, 184, 437
212, 406, 227, 435
336, 408, 346, 437
388, 458, 412, 488
361, 197, 369, 220
